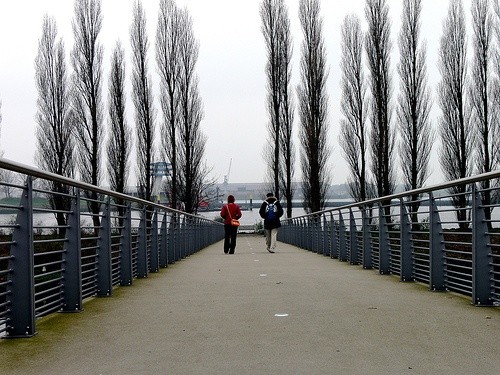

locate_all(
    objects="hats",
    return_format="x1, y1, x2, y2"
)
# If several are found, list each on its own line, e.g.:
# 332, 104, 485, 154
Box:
228, 195, 234, 203
267, 193, 273, 197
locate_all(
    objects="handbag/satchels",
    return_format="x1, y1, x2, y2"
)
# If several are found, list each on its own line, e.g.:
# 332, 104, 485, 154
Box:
231, 219, 240, 226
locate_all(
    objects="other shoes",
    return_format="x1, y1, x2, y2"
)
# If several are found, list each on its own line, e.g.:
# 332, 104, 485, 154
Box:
267, 248, 275, 253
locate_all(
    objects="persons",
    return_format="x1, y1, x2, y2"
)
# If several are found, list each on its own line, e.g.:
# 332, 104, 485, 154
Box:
259, 193, 284, 254
220, 195, 242, 254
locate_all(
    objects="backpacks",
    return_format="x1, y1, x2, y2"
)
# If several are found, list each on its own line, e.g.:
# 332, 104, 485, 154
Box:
264, 200, 279, 219
